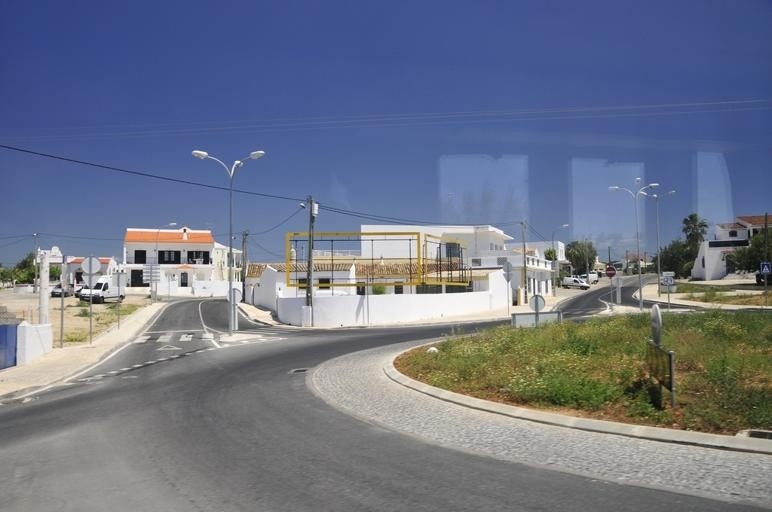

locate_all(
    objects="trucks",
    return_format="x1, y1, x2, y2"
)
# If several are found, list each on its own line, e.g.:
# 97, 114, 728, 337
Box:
579, 274, 598, 284
563, 276, 590, 290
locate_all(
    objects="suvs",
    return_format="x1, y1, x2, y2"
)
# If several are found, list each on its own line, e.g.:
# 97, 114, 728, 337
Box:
51, 283, 74, 296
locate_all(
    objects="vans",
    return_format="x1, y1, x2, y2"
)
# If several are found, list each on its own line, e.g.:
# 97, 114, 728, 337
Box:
79, 275, 126, 303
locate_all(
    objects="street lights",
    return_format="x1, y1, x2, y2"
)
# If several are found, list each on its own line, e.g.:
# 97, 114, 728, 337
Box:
154, 223, 177, 302
552, 224, 568, 296
608, 178, 676, 311
191, 150, 266, 334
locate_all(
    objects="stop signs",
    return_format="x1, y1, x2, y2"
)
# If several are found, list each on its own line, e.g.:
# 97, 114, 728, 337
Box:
606, 267, 616, 277
